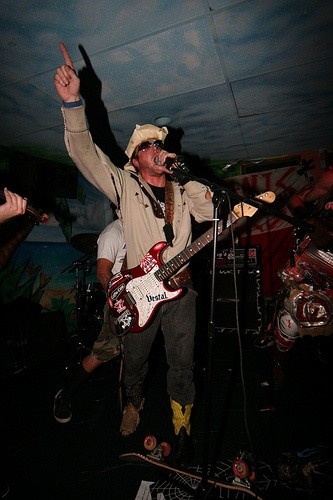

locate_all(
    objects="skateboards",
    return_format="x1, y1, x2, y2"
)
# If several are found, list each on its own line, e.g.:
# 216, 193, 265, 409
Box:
118, 435, 263, 500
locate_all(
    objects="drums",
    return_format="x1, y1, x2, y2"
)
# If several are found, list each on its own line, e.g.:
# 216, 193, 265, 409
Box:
79, 323, 101, 353
78, 282, 105, 324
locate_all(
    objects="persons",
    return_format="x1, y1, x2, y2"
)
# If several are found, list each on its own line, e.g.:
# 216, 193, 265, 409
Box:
0, 187, 27, 224
53, 43, 215, 455
53, 159, 138, 424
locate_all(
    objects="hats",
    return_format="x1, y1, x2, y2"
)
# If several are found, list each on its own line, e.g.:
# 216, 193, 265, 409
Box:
124, 123, 169, 173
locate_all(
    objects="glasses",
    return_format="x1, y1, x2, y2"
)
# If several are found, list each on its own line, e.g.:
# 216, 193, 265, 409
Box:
136, 139, 164, 163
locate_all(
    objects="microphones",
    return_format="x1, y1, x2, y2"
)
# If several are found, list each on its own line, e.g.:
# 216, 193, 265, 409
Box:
154, 155, 179, 165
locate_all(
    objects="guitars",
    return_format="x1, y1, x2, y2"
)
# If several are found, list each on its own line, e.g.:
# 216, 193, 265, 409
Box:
108, 190, 276, 333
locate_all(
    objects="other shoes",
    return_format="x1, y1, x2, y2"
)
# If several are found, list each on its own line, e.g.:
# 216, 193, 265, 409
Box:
52, 389, 72, 424
166, 424, 192, 469
120, 400, 143, 436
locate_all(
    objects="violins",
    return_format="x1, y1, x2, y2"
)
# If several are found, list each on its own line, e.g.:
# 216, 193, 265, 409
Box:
0, 188, 49, 226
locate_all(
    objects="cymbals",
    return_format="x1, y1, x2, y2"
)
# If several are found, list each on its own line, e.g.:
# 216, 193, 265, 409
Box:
69, 233, 100, 255
